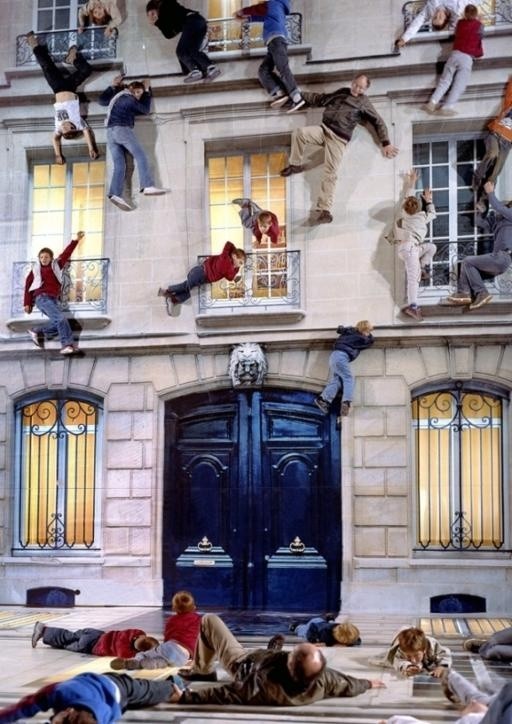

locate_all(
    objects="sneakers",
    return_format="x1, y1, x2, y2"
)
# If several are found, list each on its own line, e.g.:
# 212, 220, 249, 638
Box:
424, 102, 435, 111
207, 64, 220, 80
422, 270, 429, 279
59, 345, 80, 354
232, 199, 249, 207
441, 670, 460, 702
326, 614, 334, 621
144, 186, 165, 195
448, 292, 471, 303
340, 401, 349, 416
111, 658, 140, 670
111, 195, 132, 211
318, 211, 332, 223
166, 296, 174, 315
65, 45, 78, 64
315, 397, 329, 414
269, 94, 289, 106
158, 288, 166, 296
403, 307, 423, 320
289, 624, 296, 630
280, 166, 302, 176
184, 70, 203, 82
27, 31, 35, 45
32, 621, 44, 648
27, 329, 40, 346
464, 638, 488, 651
469, 292, 492, 309
477, 201, 485, 212
473, 175, 481, 190
439, 110, 456, 115
286, 97, 306, 114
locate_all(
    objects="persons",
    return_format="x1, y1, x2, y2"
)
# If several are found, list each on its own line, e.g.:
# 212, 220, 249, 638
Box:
157, 239, 246, 316
0, 672, 185, 724
231, 0, 308, 117
463, 627, 512, 662
393, 0, 462, 52
310, 318, 376, 420
110, 591, 202, 670
277, 72, 399, 226
21, 228, 88, 357
421, 2, 488, 117
368, 624, 453, 678
289, 614, 361, 647
96, 73, 168, 214
76, 0, 123, 37
163, 613, 388, 706
31, 621, 160, 658
385, 165, 438, 325
447, 179, 512, 314
230, 195, 282, 248
376, 668, 512, 724
143, 0, 224, 85
471, 72, 512, 213
23, 30, 100, 167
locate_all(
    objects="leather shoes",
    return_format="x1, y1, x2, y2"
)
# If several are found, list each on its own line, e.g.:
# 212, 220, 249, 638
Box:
178, 668, 217, 681
269, 635, 284, 650
